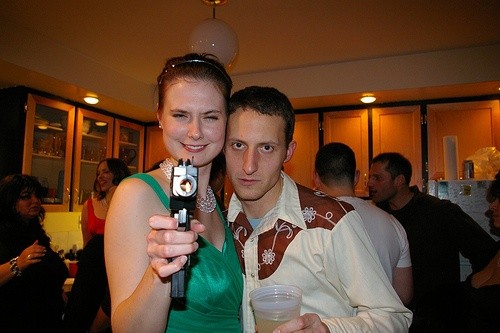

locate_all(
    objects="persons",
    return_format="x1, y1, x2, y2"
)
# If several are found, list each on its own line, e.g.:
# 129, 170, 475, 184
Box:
223, 86, 413, 333
82, 157, 132, 247
366, 153, 499, 333
104, 52, 244, 333
313, 142, 413, 307
0, 175, 70, 333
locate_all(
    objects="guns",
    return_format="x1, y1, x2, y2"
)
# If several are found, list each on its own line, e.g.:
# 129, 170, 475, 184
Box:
170, 160, 198, 298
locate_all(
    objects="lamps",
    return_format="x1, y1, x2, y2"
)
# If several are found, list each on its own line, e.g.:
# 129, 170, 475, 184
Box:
188, 0, 238, 65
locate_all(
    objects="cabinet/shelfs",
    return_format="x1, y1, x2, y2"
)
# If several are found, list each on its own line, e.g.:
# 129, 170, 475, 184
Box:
21, 93, 144, 212
284, 100, 500, 197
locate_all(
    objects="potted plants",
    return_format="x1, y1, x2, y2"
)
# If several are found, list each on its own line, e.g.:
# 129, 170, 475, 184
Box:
65, 249, 83, 278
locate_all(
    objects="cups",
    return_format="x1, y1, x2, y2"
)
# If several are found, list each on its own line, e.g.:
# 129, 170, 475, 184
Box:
249, 284, 303, 333
443, 135, 457, 180
68, 261, 79, 278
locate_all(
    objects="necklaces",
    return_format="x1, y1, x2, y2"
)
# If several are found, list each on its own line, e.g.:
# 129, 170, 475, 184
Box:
158, 158, 216, 213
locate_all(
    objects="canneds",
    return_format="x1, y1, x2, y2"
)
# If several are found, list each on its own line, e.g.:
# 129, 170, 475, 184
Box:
463, 159, 474, 180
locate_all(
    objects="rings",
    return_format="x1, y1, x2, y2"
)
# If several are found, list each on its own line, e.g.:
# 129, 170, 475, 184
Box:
28, 255, 31, 259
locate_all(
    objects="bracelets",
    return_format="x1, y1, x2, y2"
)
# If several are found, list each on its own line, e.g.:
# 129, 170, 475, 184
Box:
10, 256, 25, 277
